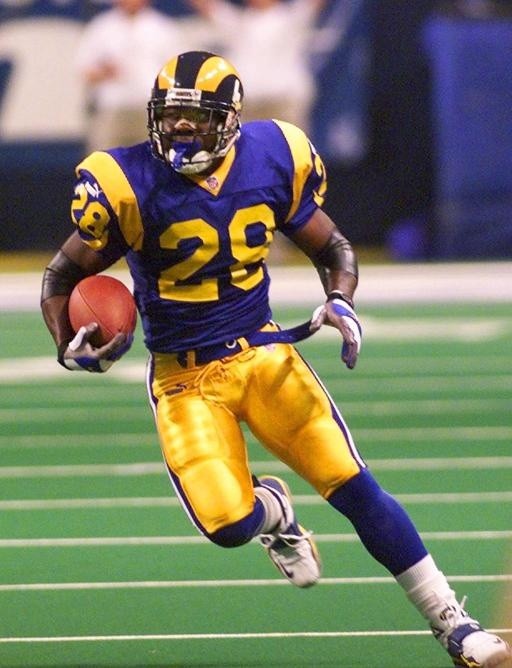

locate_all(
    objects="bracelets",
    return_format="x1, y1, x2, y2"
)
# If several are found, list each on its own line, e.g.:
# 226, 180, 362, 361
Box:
326, 292, 355, 310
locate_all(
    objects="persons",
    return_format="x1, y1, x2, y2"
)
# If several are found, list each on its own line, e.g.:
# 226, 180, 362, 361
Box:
187, 1, 325, 136
171, 1, 248, 52
39, 49, 510, 667
70, 1, 192, 155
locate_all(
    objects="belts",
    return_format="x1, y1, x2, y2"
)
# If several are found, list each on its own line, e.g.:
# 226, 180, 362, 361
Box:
197, 318, 317, 366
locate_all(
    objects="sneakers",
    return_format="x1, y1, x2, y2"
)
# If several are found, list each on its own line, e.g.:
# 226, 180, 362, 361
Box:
429, 593, 512, 668
251, 473, 323, 590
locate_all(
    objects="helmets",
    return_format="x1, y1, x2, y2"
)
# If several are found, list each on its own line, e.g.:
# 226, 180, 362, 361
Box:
144, 47, 247, 178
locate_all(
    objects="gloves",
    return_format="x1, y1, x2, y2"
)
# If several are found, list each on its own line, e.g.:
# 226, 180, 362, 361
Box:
308, 289, 362, 372
51, 322, 134, 376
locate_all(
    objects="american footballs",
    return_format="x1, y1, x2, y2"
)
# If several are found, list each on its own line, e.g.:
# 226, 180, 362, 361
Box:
69, 275, 136, 348
